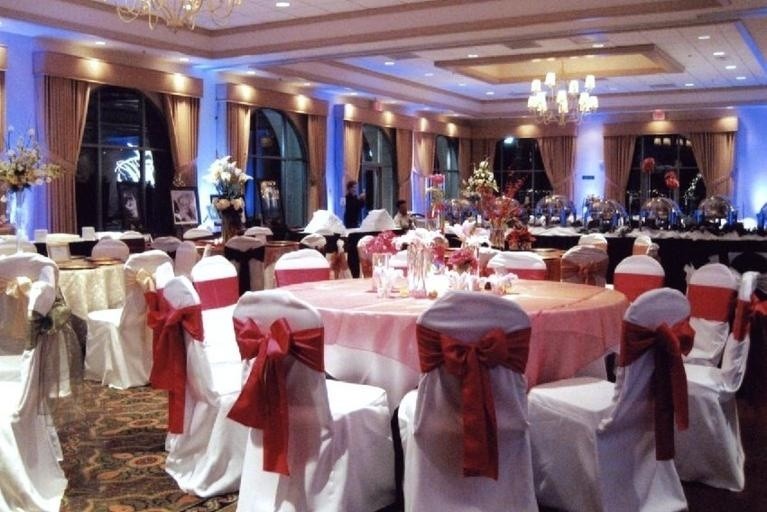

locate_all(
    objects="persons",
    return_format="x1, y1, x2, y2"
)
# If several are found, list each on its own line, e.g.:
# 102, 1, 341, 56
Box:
122, 188, 149, 231
344, 179, 368, 227
393, 198, 417, 227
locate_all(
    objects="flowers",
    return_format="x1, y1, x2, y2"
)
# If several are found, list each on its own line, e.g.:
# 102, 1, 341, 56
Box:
460, 159, 499, 202
640, 157, 655, 176
663, 170, 680, 191
0, 125, 62, 226
202, 155, 254, 210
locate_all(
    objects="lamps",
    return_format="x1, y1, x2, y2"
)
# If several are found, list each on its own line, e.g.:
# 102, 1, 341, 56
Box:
527, 58, 600, 127
115, 0, 243, 32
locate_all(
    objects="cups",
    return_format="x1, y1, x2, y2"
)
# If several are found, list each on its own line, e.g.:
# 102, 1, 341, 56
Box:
407, 245, 435, 299
34, 228, 48, 242
82, 226, 95, 238
371, 253, 394, 300
